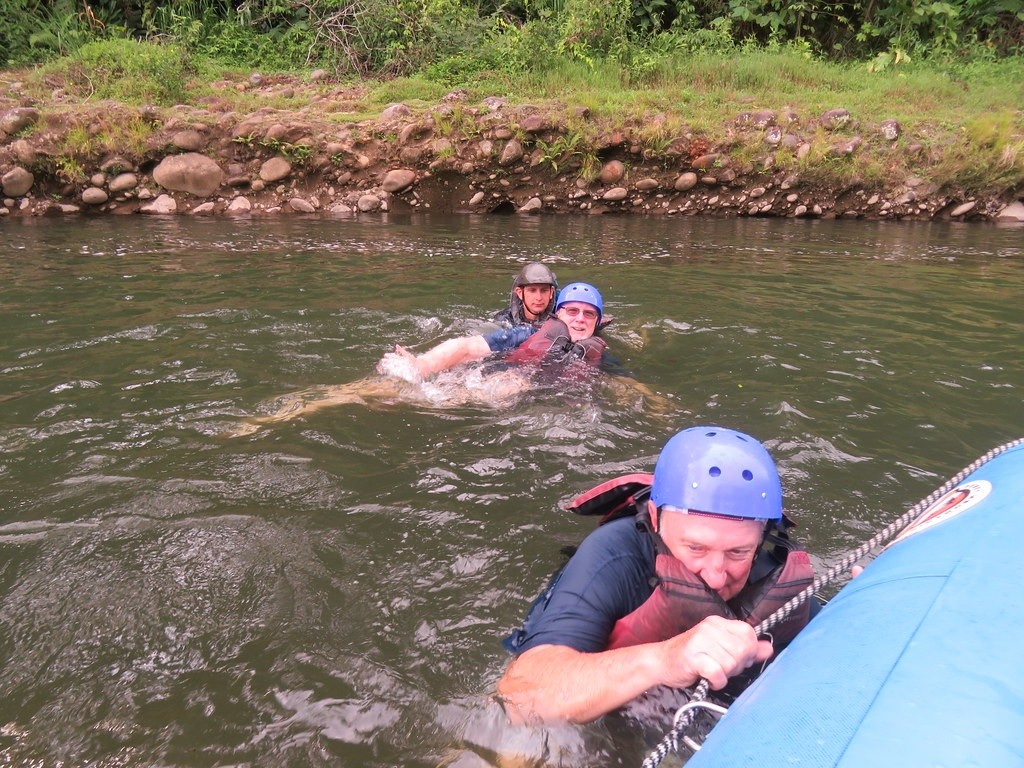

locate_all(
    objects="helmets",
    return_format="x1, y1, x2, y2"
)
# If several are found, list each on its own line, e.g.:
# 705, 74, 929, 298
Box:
516, 264, 555, 286
650, 426, 782, 524
555, 283, 603, 327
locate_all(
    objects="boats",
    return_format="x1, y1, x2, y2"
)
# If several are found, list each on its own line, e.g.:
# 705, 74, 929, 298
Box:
640, 438, 1024, 768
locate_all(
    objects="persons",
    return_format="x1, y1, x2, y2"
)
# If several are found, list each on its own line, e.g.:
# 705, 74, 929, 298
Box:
496, 263, 562, 323
501, 424, 863, 725
387, 281, 606, 369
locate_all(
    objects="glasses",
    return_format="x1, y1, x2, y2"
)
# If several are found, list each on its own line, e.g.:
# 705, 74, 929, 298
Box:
560, 307, 599, 319
